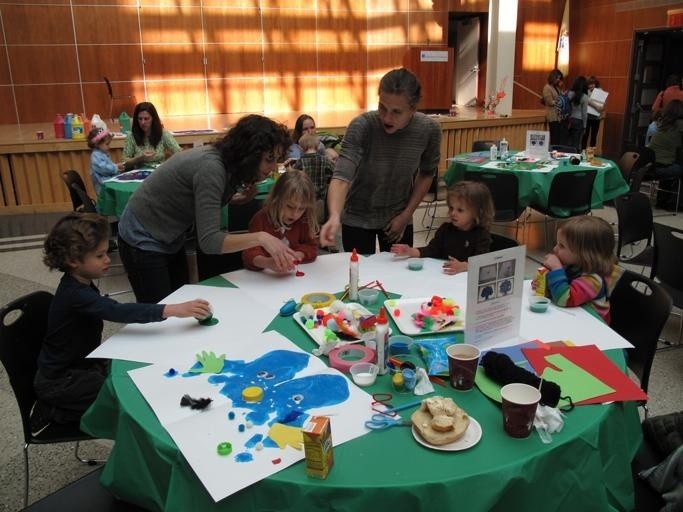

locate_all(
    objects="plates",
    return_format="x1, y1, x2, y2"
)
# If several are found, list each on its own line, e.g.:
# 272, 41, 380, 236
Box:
294, 301, 392, 349
411, 415, 481, 451
383, 296, 464, 335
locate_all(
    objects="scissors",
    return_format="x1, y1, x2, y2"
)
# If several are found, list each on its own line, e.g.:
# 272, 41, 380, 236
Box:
365, 414, 412, 431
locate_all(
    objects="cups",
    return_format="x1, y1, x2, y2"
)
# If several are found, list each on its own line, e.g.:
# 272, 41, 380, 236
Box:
358, 288, 378, 304
500, 382, 541, 438
445, 342, 480, 391
35, 132, 44, 141
388, 335, 414, 356
527, 297, 550, 312
407, 257, 424, 271
385, 354, 433, 400
349, 362, 378, 385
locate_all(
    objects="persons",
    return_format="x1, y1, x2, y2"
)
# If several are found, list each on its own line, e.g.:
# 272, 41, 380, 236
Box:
35, 67, 682, 430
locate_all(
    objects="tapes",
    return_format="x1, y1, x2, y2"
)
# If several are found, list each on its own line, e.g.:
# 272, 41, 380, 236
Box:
302, 292, 337, 309
330, 345, 375, 371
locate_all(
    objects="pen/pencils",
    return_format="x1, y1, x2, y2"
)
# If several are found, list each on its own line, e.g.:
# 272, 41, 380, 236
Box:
339, 280, 388, 301
384, 401, 421, 413
389, 357, 447, 386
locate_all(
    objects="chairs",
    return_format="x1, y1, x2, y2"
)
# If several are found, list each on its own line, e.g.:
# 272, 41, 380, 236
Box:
102, 75, 136, 119
0, 291, 109, 512
59, 169, 117, 223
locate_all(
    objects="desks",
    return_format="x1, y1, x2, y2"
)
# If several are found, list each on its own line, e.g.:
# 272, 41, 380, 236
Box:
102, 162, 280, 284
109, 251, 626, 512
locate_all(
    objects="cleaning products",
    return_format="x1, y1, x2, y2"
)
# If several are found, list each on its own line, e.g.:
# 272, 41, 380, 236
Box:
348, 248, 359, 300
490, 143, 498, 161
500, 137, 508, 156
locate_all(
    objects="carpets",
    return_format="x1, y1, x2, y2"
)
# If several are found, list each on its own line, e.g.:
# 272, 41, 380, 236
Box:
19, 464, 148, 512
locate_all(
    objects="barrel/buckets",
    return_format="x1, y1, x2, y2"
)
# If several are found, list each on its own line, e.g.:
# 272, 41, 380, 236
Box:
54, 113, 65, 138
119, 111, 131, 132
71, 114, 89, 139
64, 112, 73, 139
620, 151, 639, 175
91, 114, 107, 132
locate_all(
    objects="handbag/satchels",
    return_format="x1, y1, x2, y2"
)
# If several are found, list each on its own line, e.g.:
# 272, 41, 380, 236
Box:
644, 90, 665, 148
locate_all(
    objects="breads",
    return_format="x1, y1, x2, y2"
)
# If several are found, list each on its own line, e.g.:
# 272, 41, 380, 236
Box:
410, 394, 471, 447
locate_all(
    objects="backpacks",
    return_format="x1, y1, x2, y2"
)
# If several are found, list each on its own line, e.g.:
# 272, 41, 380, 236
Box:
553, 89, 573, 121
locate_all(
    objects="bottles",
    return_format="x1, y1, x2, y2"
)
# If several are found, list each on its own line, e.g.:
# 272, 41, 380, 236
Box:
53, 114, 64, 138
63, 113, 73, 139
83, 118, 89, 137
117, 111, 129, 133
488, 138, 609, 174
347, 247, 359, 301
